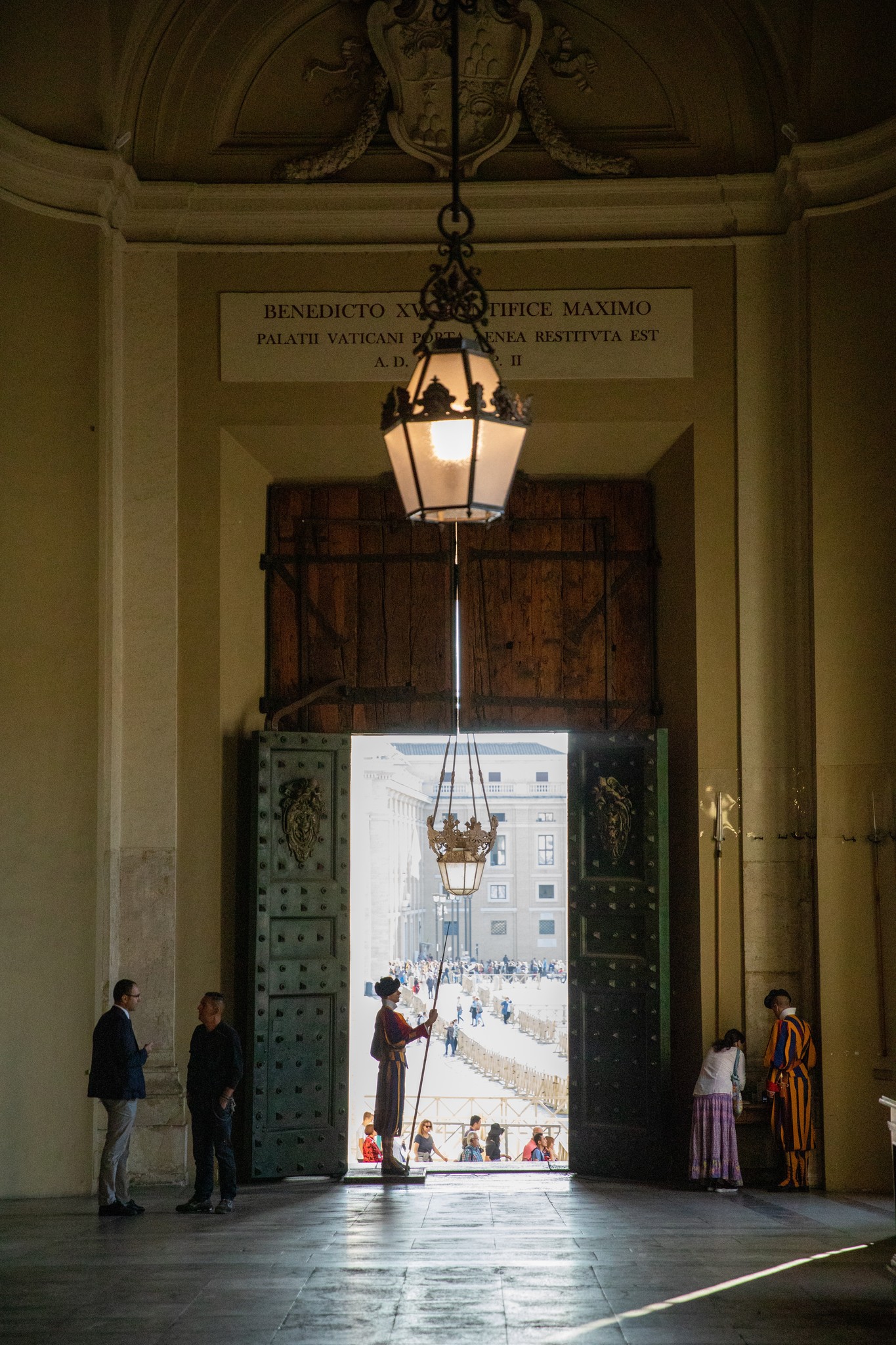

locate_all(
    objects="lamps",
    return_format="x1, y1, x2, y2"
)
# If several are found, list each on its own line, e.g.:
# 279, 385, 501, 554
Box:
378, 0, 533, 532
426, 731, 499, 898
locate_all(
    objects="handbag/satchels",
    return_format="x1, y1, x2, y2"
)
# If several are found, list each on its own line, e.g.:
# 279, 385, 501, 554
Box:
732, 1049, 742, 1119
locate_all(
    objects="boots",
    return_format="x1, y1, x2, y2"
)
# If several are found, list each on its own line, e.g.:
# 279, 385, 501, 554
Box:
394, 1157, 411, 1171
796, 1150, 809, 1192
381, 1135, 409, 1175
770, 1150, 800, 1192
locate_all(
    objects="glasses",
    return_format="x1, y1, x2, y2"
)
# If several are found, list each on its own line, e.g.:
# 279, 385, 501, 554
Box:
533, 1132, 539, 1134
125, 993, 140, 998
423, 1124, 432, 1128
471, 1137, 478, 1139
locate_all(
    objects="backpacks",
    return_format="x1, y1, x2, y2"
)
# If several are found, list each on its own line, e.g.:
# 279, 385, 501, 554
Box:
459, 1147, 473, 1162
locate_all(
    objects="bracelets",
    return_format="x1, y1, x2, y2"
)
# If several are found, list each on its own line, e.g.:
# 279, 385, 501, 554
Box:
220, 1093, 230, 1102
505, 1154, 506, 1157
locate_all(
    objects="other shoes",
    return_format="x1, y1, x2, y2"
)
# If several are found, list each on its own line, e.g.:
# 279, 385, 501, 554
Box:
176, 1196, 214, 1212
444, 1050, 457, 1056
416, 1040, 434, 1045
116, 1200, 146, 1213
715, 1182, 738, 1192
707, 1180, 715, 1191
214, 1199, 236, 1214
98, 1200, 137, 1217
457, 1020, 485, 1027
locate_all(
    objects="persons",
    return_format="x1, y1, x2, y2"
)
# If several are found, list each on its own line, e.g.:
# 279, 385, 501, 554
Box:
370, 976, 440, 1175
388, 952, 568, 1058
689, 1028, 746, 1191
462, 1114, 559, 1161
87, 979, 154, 1217
362, 1124, 383, 1162
764, 989, 818, 1193
459, 1136, 469, 1162
356, 1112, 374, 1163
392, 1136, 406, 1162
175, 991, 245, 1215
414, 1119, 448, 1162
462, 1130, 483, 1162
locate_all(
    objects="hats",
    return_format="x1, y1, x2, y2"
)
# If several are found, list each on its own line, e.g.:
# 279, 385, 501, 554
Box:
418, 1012, 423, 1015
764, 988, 791, 1008
375, 977, 401, 996
488, 1123, 505, 1136
